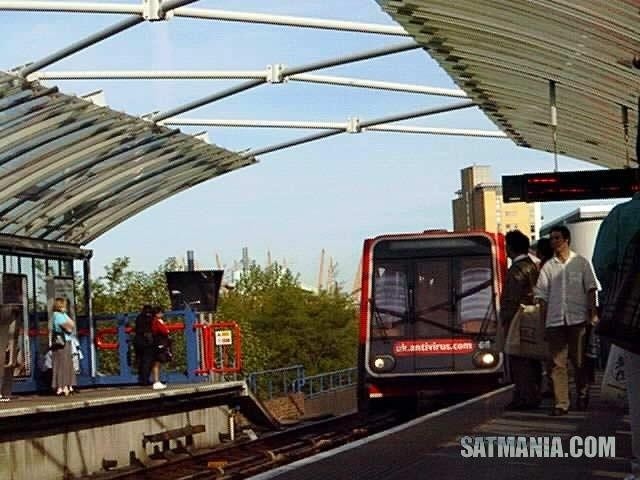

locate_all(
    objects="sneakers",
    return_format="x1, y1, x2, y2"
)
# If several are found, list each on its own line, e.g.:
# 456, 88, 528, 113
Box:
549, 402, 571, 417
152, 382, 167, 390
577, 395, 589, 407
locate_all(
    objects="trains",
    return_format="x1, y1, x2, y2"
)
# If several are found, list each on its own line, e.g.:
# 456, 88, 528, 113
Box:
357, 230, 507, 417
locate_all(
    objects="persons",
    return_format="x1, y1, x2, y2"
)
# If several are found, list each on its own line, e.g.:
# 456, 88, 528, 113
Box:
535, 236, 571, 398
150, 306, 175, 391
498, 230, 541, 412
0, 303, 25, 403
528, 223, 597, 417
48, 296, 77, 399
592, 170, 639, 479
133, 304, 170, 387
579, 309, 602, 386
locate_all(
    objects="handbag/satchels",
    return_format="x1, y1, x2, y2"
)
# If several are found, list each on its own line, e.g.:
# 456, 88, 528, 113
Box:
503, 300, 550, 358
53, 332, 66, 349
